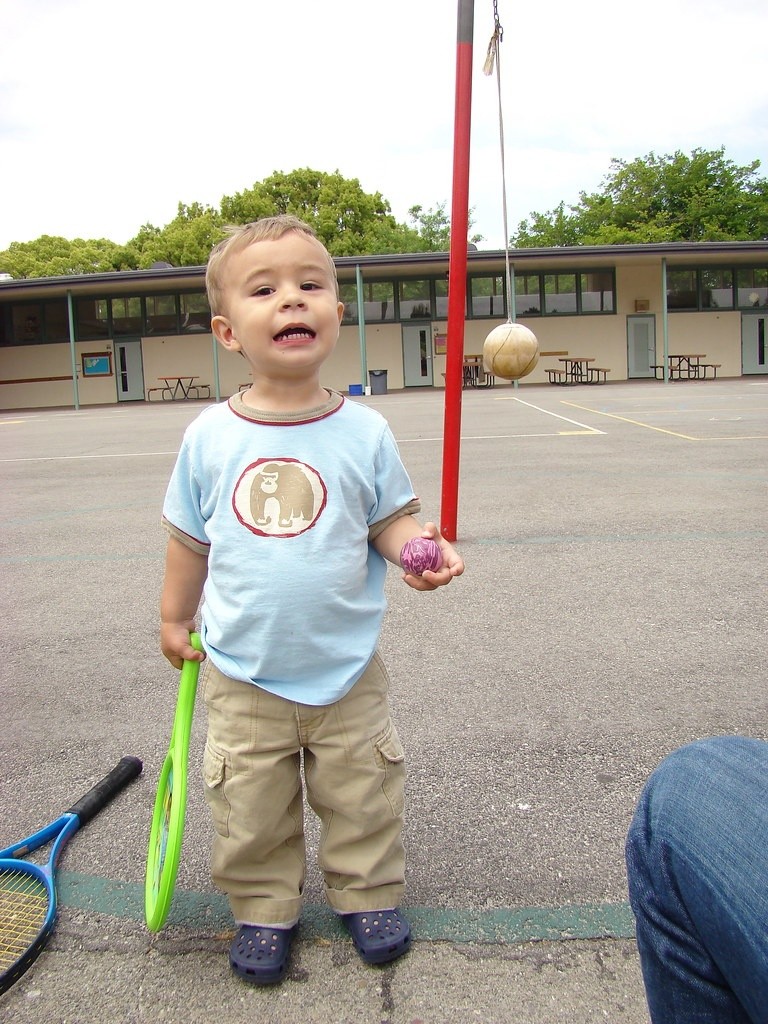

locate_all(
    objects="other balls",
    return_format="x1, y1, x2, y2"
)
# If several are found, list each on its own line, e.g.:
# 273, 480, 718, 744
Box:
481, 323, 540, 379
397, 536, 447, 574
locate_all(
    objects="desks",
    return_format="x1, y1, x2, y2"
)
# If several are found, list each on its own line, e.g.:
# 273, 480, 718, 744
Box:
462, 362, 482, 391
157, 376, 200, 400
663, 354, 707, 381
558, 358, 595, 386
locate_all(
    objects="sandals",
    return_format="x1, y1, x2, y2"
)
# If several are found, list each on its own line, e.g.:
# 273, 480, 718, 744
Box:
229, 923, 297, 983
342, 909, 411, 964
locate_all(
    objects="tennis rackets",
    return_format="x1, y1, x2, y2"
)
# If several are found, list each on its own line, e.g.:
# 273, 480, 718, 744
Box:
1, 756, 143, 998
142, 629, 201, 933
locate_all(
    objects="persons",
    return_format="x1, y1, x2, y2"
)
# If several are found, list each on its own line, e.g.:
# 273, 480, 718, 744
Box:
160, 217, 463, 987
626, 737, 768, 1024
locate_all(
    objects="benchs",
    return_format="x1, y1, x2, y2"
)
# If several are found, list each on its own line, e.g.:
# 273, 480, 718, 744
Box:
484, 371, 497, 389
238, 382, 253, 393
441, 372, 447, 381
588, 367, 611, 383
691, 364, 721, 380
145, 386, 174, 402
186, 384, 212, 400
544, 368, 566, 385
650, 365, 678, 380
